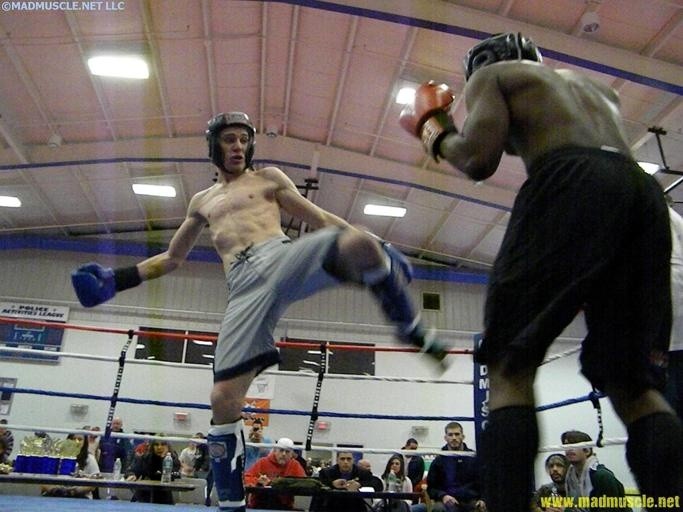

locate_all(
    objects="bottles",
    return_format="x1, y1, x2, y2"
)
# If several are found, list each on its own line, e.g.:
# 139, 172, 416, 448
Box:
550, 488, 561, 504
160, 452, 172, 485
388, 469, 395, 493
110, 457, 120, 479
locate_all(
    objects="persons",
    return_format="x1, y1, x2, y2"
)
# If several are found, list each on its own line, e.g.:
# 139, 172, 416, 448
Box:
530, 454, 570, 512
667, 207, 683, 419
71, 111, 456, 512
399, 32, 683, 512
39, 417, 489, 512
0, 419, 14, 463
546, 430, 633, 512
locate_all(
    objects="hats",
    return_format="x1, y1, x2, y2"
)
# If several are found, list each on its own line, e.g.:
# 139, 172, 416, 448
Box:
273, 437, 295, 452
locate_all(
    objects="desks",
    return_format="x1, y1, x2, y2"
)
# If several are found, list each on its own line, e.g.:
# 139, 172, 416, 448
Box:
0, 472, 439, 511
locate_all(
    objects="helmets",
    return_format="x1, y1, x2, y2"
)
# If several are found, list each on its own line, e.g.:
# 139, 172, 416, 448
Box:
204, 109, 257, 171
459, 29, 543, 85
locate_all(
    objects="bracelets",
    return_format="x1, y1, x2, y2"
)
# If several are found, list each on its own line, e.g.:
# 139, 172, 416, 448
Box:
115, 265, 141, 291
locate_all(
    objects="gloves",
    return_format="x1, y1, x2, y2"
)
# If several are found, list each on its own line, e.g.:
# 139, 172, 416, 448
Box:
70, 262, 116, 309
397, 76, 456, 135
